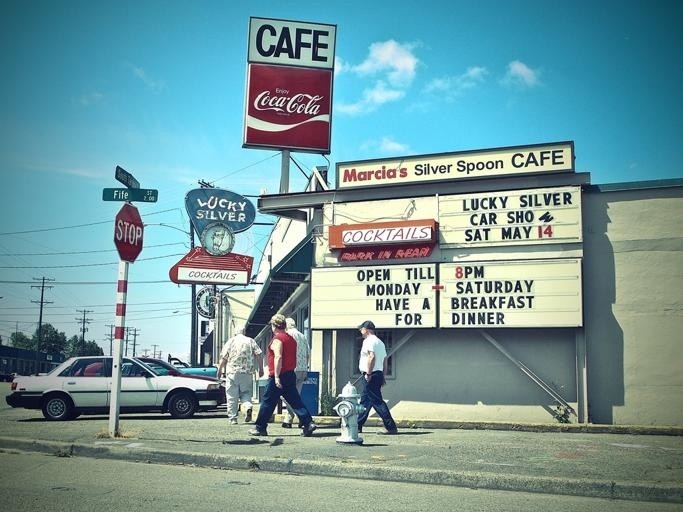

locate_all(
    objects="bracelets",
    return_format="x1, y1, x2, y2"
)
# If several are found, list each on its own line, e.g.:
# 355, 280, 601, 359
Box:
366, 373, 371, 376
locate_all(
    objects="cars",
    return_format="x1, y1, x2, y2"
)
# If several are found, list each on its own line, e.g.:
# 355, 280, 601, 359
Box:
6, 355, 227, 422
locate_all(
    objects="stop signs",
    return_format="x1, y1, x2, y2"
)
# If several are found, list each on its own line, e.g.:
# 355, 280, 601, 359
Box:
113, 204, 144, 262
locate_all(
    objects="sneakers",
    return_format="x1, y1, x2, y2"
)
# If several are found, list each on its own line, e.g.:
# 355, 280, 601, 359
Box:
281, 421, 292, 428
247, 427, 268, 436
300, 422, 317, 437
228, 417, 238, 424
376, 427, 398, 434
244, 409, 252, 422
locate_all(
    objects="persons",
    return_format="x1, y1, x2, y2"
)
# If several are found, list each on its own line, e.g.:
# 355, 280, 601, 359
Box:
279, 317, 308, 429
352, 320, 398, 435
242, 313, 317, 436
215, 325, 262, 425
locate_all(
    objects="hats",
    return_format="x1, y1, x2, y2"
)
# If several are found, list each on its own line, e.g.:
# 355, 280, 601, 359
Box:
358, 320, 376, 330
266, 313, 287, 330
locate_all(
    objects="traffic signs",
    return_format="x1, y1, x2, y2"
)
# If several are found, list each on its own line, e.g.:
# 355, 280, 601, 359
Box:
102, 165, 158, 203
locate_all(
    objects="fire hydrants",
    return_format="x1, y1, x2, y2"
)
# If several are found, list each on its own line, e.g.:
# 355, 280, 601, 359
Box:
332, 381, 367, 445
